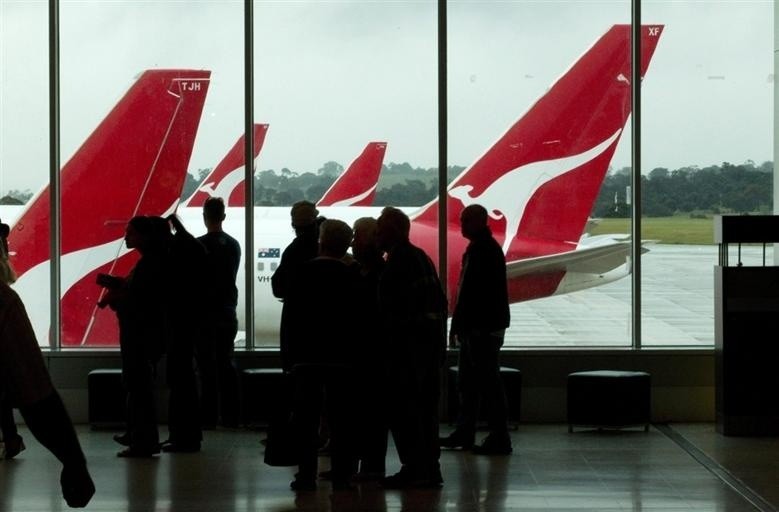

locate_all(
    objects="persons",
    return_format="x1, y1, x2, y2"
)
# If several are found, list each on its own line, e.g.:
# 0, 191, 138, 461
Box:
438, 205, 512, 456
271, 200, 449, 492
0, 279, 95, 508
96, 198, 241, 456
0, 220, 26, 462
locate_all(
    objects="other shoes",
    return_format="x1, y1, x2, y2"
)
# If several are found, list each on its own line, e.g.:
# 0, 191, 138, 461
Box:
440, 424, 475, 450
471, 425, 512, 456
118, 447, 153, 458
113, 429, 132, 445
159, 432, 203, 452
260, 423, 444, 493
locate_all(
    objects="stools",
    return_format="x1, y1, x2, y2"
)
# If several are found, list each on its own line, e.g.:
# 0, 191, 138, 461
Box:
88, 366, 138, 432
444, 367, 523, 433
563, 368, 650, 436
239, 367, 284, 426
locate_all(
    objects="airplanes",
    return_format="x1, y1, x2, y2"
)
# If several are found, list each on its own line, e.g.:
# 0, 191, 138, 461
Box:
0, 24, 663, 346
